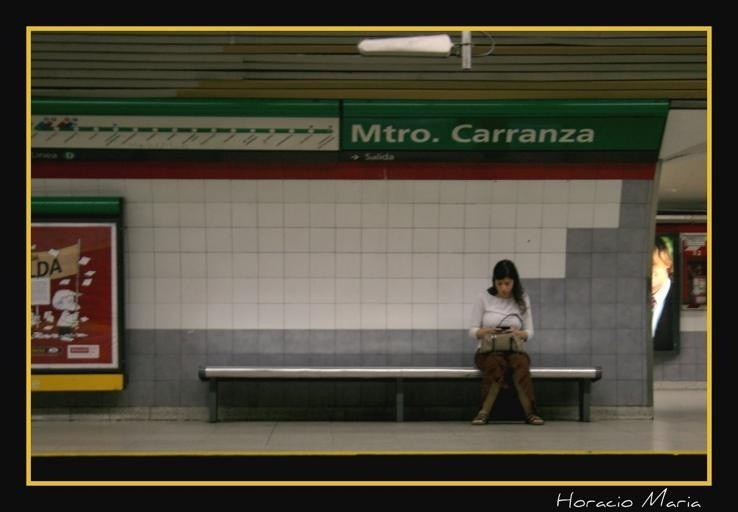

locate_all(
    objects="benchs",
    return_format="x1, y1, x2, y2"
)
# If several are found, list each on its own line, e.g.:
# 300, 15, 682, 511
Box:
197, 363, 604, 423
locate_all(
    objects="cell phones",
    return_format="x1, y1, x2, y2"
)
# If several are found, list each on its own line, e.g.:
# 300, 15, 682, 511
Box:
496, 326, 510, 331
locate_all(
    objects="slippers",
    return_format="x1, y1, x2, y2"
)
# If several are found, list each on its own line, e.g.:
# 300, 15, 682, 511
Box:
526, 414, 544, 424
472, 414, 489, 425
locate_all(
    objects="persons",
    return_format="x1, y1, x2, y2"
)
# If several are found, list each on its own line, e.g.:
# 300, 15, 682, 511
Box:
652, 236, 679, 338
467, 258, 545, 424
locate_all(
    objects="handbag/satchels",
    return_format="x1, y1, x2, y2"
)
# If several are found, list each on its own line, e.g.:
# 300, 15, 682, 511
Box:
480, 333, 526, 354
